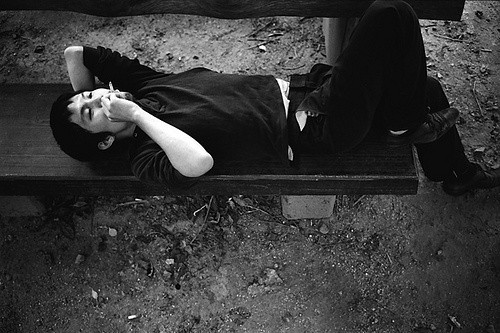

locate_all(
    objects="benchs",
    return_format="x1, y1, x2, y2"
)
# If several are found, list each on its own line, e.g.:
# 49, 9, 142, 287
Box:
1, 0, 465, 195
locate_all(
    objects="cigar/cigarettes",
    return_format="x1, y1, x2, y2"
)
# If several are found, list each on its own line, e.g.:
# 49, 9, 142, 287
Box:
109, 80, 115, 92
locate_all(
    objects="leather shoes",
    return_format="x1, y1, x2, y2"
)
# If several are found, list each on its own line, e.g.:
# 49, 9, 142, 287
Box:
402, 108, 459, 144
443, 164, 500, 196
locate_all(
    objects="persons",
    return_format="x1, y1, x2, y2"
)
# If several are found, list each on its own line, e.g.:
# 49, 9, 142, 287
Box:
50, 1, 499, 195
